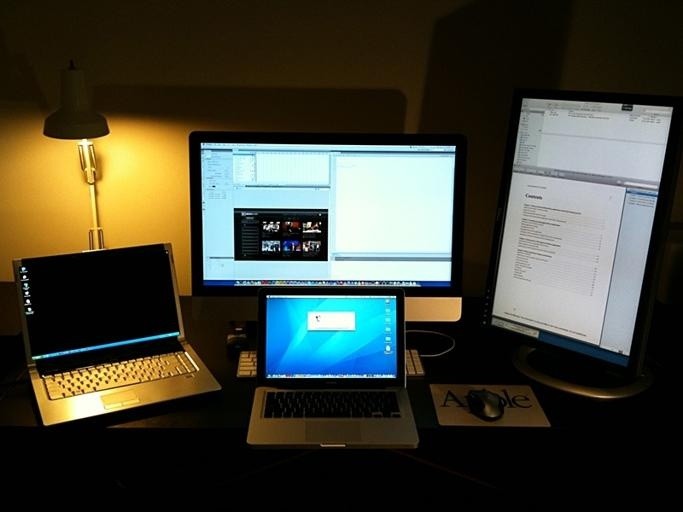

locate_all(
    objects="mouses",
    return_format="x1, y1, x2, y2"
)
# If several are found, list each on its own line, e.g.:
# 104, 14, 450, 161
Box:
466, 388, 504, 421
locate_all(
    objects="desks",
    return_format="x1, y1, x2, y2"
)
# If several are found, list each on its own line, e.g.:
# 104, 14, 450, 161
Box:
0, 280, 682, 512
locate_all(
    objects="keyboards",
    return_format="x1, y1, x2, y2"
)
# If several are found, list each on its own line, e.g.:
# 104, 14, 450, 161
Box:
235, 348, 426, 378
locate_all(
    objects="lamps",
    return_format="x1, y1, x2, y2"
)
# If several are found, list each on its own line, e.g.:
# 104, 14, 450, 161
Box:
43, 58, 109, 251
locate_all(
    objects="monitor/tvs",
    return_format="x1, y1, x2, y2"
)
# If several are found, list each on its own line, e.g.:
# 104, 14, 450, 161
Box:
187, 130, 466, 323
479, 88, 682, 399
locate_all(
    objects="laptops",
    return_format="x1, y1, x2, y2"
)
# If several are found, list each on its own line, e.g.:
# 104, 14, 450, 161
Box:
12, 242, 222, 427
245, 287, 421, 449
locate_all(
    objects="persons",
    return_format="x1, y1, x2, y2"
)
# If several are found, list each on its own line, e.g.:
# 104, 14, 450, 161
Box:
260, 219, 322, 254
